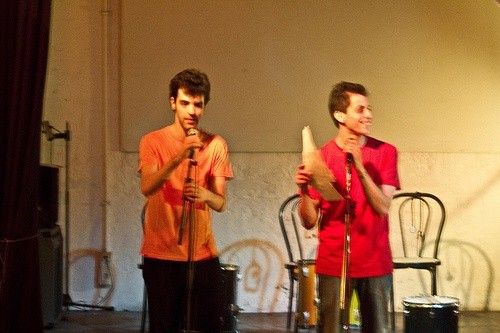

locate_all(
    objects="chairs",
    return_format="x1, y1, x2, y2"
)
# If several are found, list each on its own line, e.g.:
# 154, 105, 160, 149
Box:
278, 193, 334, 333
390, 191, 446, 333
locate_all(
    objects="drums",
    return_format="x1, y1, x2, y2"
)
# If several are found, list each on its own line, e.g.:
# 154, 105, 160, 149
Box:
402, 293, 461, 333
219, 265, 243, 333
292, 256, 362, 331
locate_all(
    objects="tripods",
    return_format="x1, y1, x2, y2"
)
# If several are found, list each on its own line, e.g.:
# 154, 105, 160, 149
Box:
64, 120, 115, 312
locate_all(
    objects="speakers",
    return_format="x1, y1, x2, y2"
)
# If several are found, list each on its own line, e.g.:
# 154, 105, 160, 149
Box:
37, 225, 63, 328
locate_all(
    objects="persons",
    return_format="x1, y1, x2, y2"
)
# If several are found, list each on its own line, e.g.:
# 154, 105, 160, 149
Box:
294, 81, 401, 333
138, 69, 234, 333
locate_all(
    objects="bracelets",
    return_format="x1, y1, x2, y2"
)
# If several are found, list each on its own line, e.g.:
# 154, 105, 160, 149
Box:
359, 170, 368, 178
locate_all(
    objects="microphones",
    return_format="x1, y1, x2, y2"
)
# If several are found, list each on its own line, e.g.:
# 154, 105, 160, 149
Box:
187, 128, 198, 159
345, 138, 355, 162
43, 120, 53, 141
